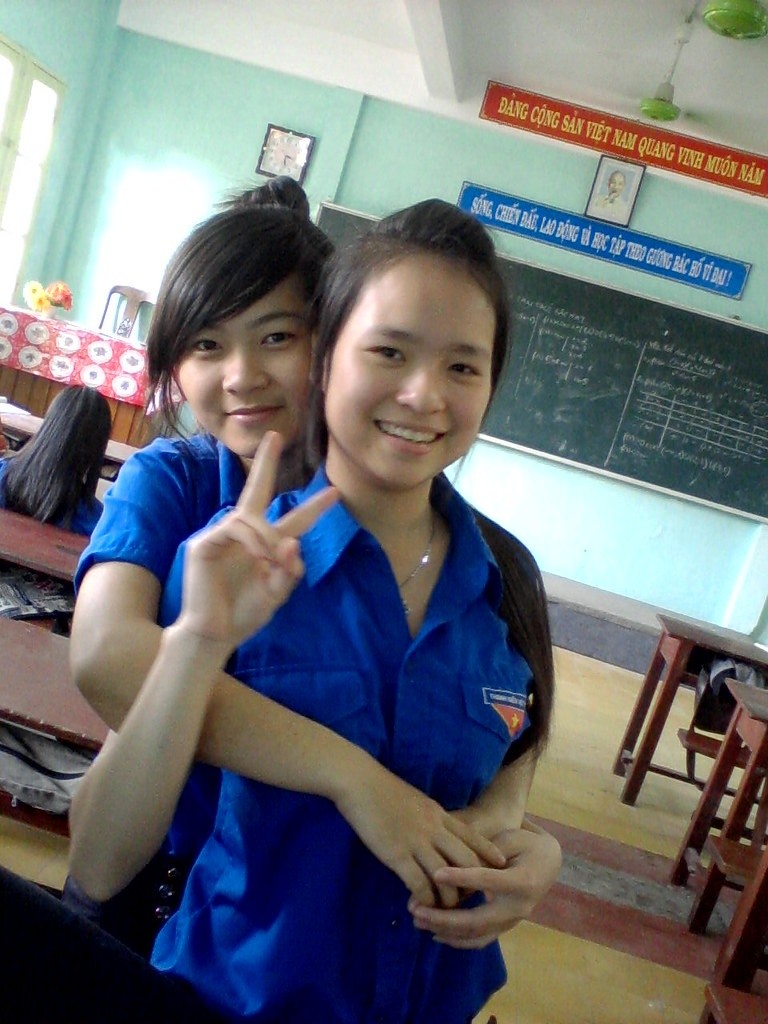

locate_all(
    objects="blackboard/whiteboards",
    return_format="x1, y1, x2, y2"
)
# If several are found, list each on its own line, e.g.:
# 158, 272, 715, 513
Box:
313, 200, 767, 528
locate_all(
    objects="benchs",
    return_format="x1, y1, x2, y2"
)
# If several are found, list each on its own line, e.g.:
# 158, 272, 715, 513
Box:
708, 834, 766, 880
677, 729, 765, 779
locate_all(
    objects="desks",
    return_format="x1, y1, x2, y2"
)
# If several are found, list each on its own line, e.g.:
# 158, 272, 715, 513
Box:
615, 613, 768, 807
669, 679, 768, 931
0, 304, 186, 450
0, 399, 151, 835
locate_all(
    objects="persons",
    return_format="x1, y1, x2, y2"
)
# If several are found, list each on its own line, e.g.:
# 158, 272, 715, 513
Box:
0, 383, 111, 536
593, 171, 630, 221
72, 179, 563, 958
67, 201, 547, 1024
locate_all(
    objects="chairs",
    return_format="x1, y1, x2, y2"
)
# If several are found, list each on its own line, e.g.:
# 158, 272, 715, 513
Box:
99, 286, 159, 344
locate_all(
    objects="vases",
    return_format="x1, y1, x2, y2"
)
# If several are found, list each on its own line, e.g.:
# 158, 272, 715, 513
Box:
40, 307, 58, 320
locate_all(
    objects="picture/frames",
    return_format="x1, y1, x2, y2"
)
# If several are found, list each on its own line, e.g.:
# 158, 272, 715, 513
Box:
584, 154, 646, 229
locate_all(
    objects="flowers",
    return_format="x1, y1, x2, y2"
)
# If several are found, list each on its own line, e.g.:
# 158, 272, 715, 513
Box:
24, 280, 73, 312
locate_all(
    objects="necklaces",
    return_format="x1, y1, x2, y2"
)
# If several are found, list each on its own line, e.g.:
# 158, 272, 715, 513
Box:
397, 517, 436, 617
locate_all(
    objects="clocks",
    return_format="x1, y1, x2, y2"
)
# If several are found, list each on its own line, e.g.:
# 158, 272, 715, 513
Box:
255, 122, 316, 188
